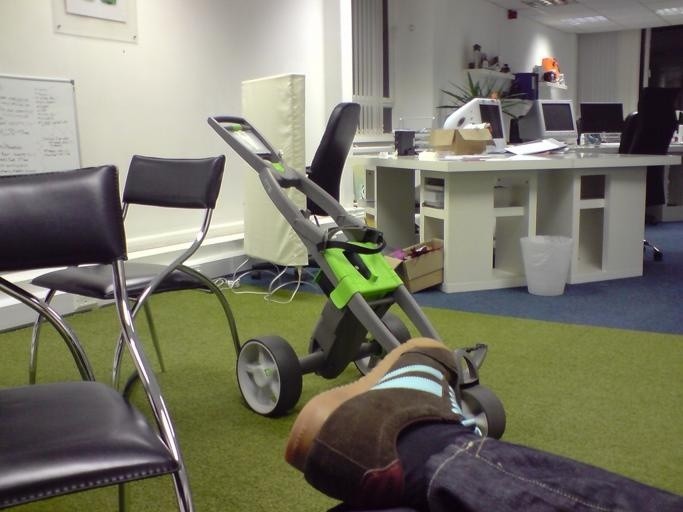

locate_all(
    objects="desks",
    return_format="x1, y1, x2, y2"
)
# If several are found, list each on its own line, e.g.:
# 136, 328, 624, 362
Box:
360, 140, 683, 296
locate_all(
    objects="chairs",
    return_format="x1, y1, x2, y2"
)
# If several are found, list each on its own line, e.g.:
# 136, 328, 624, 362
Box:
304, 101, 361, 215
0, 164, 199, 512
618, 87, 680, 261
28, 153, 244, 415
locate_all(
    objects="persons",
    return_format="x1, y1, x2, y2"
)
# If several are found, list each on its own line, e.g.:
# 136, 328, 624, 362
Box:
284, 335, 683, 511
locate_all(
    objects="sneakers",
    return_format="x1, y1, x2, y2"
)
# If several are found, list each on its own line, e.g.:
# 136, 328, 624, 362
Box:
281, 335, 483, 510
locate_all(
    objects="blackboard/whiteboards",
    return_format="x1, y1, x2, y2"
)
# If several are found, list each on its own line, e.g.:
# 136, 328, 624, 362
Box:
0, 76, 82, 175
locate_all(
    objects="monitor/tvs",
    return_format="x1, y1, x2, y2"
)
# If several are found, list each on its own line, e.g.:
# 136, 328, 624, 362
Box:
519, 99, 578, 141
579, 102, 624, 132
444, 98, 506, 152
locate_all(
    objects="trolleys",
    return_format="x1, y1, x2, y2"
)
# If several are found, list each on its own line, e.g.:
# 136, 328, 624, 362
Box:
207, 114, 506, 440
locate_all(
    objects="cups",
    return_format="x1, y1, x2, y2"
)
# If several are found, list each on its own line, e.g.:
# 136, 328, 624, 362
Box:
394, 129, 416, 156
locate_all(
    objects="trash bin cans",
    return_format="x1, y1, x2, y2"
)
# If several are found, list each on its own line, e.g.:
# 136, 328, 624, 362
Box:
520, 235, 573, 296
